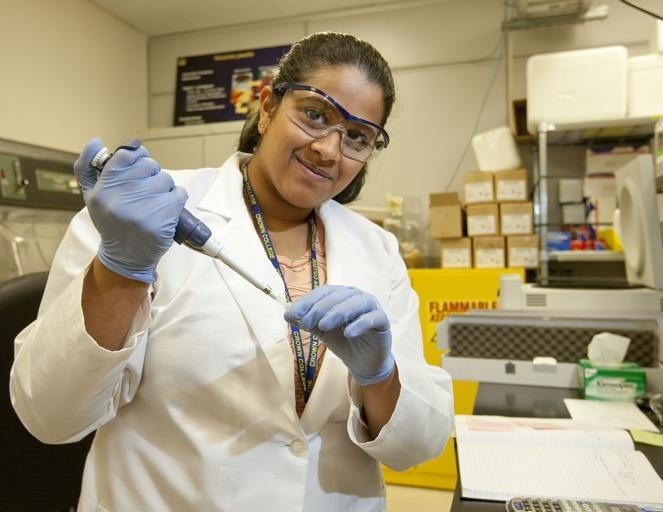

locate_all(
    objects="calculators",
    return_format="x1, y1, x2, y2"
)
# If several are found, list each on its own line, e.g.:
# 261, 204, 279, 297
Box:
505, 497, 663, 512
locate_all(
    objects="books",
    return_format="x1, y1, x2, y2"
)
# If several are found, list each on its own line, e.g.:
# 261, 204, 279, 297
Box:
450, 413, 663, 512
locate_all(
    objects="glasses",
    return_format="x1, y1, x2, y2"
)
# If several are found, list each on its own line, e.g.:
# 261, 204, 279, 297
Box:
271, 79, 390, 165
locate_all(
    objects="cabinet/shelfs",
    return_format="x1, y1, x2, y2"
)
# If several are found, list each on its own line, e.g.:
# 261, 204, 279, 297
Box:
533, 121, 663, 288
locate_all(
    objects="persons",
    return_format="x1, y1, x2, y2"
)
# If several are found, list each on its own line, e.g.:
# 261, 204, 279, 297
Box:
7, 30, 455, 512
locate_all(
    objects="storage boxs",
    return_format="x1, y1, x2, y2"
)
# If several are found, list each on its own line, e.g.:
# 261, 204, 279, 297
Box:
428, 166, 540, 270
